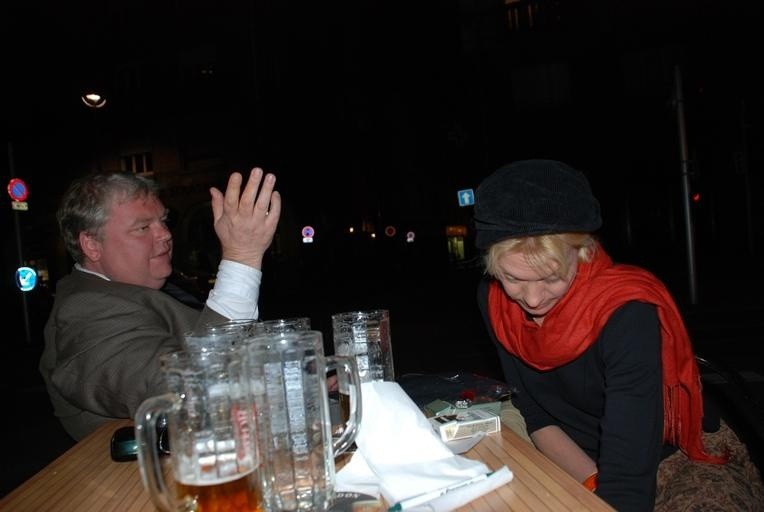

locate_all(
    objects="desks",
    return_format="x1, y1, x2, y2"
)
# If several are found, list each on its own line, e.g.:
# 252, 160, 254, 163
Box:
1, 404, 616, 512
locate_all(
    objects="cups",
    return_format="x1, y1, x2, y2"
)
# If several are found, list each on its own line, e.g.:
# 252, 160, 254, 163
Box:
331, 307, 398, 411
132, 313, 363, 511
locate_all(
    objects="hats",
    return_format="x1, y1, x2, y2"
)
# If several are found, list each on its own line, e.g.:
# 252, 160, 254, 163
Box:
467, 159, 603, 246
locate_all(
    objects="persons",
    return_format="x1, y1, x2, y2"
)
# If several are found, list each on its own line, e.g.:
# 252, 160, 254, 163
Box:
472, 154, 763, 511
40, 163, 340, 444
